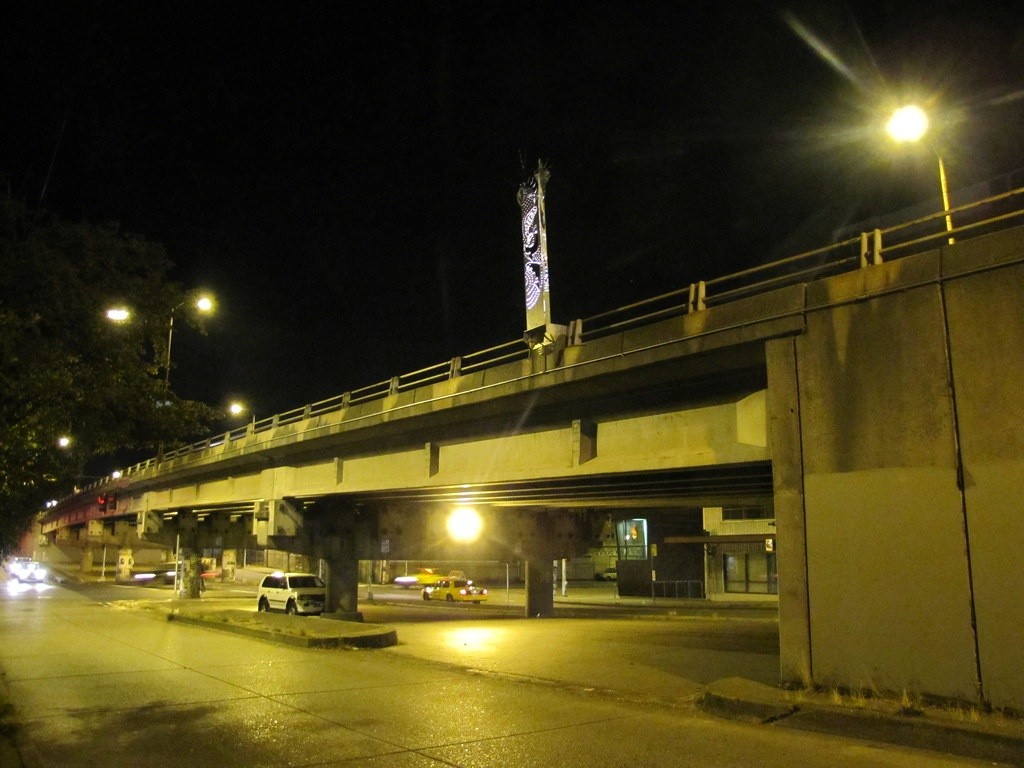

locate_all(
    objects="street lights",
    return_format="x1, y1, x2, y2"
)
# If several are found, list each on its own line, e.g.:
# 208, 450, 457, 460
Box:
883, 102, 955, 241
104, 289, 216, 457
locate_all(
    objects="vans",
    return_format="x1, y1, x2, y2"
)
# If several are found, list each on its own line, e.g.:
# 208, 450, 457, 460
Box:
255, 572, 327, 616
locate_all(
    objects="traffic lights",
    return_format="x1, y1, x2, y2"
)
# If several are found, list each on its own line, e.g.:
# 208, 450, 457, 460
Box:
108, 492, 117, 509
98, 492, 106, 512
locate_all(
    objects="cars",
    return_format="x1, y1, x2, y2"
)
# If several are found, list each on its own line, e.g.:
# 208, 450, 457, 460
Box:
395, 568, 468, 590
422, 570, 488, 604
596, 566, 617, 581
8, 556, 47, 584
131, 559, 210, 586
